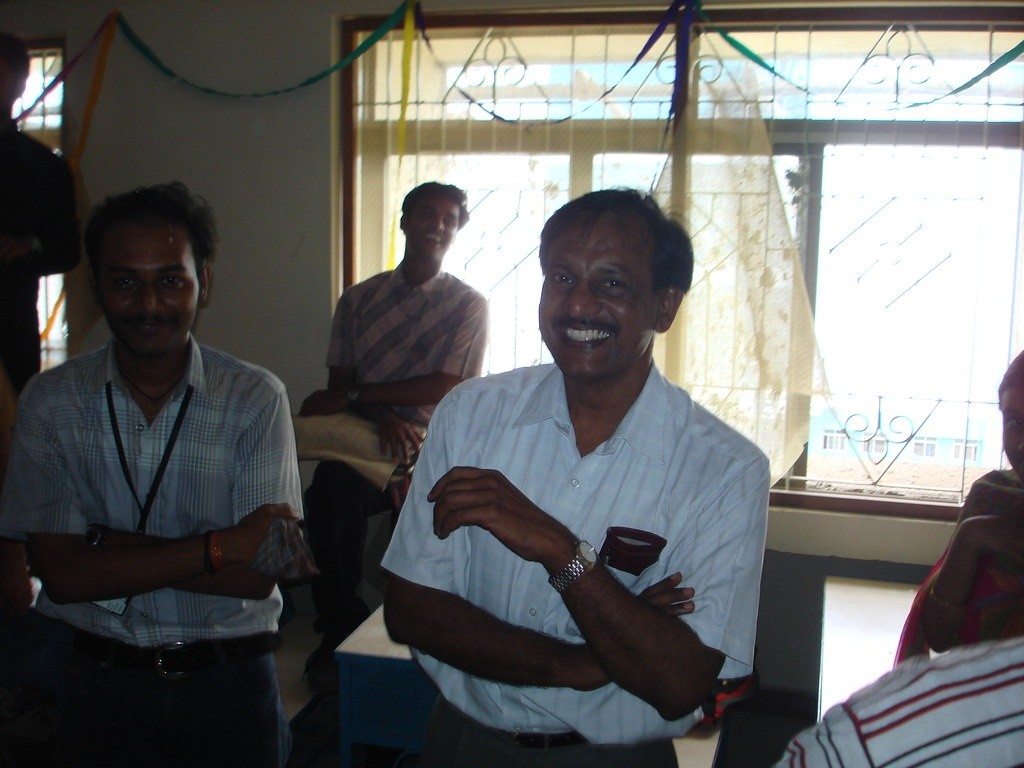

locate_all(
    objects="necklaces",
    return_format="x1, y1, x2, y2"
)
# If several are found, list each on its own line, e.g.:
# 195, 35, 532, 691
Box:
119, 370, 183, 416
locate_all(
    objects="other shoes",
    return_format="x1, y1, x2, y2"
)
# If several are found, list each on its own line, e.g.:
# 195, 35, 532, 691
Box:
302, 627, 354, 691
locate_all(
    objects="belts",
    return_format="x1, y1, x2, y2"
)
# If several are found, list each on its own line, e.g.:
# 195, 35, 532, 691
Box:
72, 630, 263, 683
501, 727, 587, 750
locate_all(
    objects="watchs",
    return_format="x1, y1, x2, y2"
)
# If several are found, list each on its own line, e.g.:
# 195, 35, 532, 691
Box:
548, 540, 601, 594
346, 385, 359, 409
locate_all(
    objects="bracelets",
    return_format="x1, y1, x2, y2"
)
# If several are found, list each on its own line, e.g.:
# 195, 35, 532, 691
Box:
930, 586, 970, 610
202, 530, 223, 575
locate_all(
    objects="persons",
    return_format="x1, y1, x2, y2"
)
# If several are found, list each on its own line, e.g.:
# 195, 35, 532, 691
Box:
0, 29, 82, 396
890, 350, 1024, 668
0, 183, 306, 768
379, 187, 770, 768
297, 182, 490, 690
